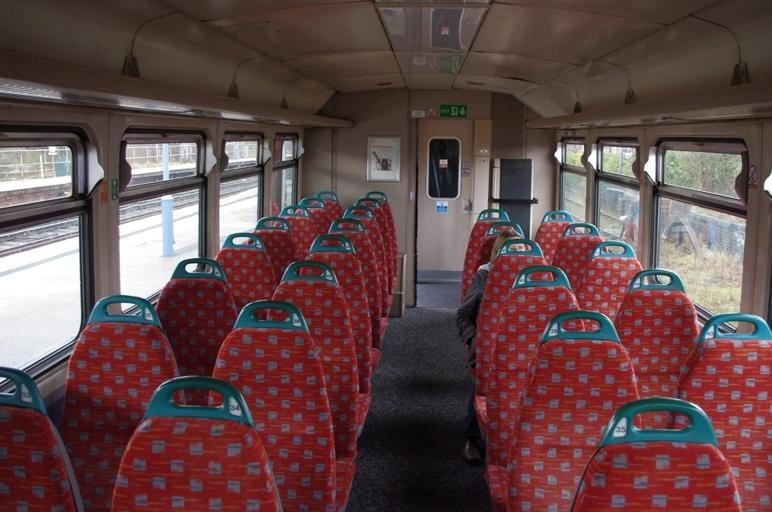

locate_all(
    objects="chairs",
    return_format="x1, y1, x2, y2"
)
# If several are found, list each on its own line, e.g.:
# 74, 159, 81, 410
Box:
473, 239, 555, 441
267, 260, 360, 511
157, 259, 238, 405
328, 189, 399, 370
506, 310, 640, 512
462, 210, 512, 298
614, 269, 701, 430
486, 264, 586, 512
534, 210, 574, 264
109, 375, 283, 511
62, 294, 185, 511
0, 367, 84, 511
276, 191, 344, 259
666, 313, 771, 511
216, 232, 278, 314
569, 396, 741, 511
208, 300, 336, 512
552, 223, 608, 299
475, 222, 527, 275
247, 217, 295, 280
301, 233, 372, 437
578, 241, 643, 326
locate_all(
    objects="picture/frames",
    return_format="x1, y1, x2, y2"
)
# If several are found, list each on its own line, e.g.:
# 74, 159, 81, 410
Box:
367, 135, 401, 182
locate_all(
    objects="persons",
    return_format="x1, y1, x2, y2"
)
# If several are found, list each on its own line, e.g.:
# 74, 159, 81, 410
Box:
477, 231, 526, 272
456, 268, 490, 466
